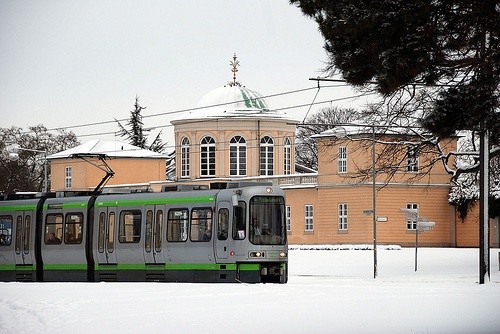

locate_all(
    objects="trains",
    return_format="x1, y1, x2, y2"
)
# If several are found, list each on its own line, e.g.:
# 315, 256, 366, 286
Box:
0, 152, 290, 284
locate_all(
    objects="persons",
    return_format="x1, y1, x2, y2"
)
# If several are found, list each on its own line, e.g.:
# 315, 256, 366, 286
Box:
46, 232, 83, 245
0, 233, 6, 246
